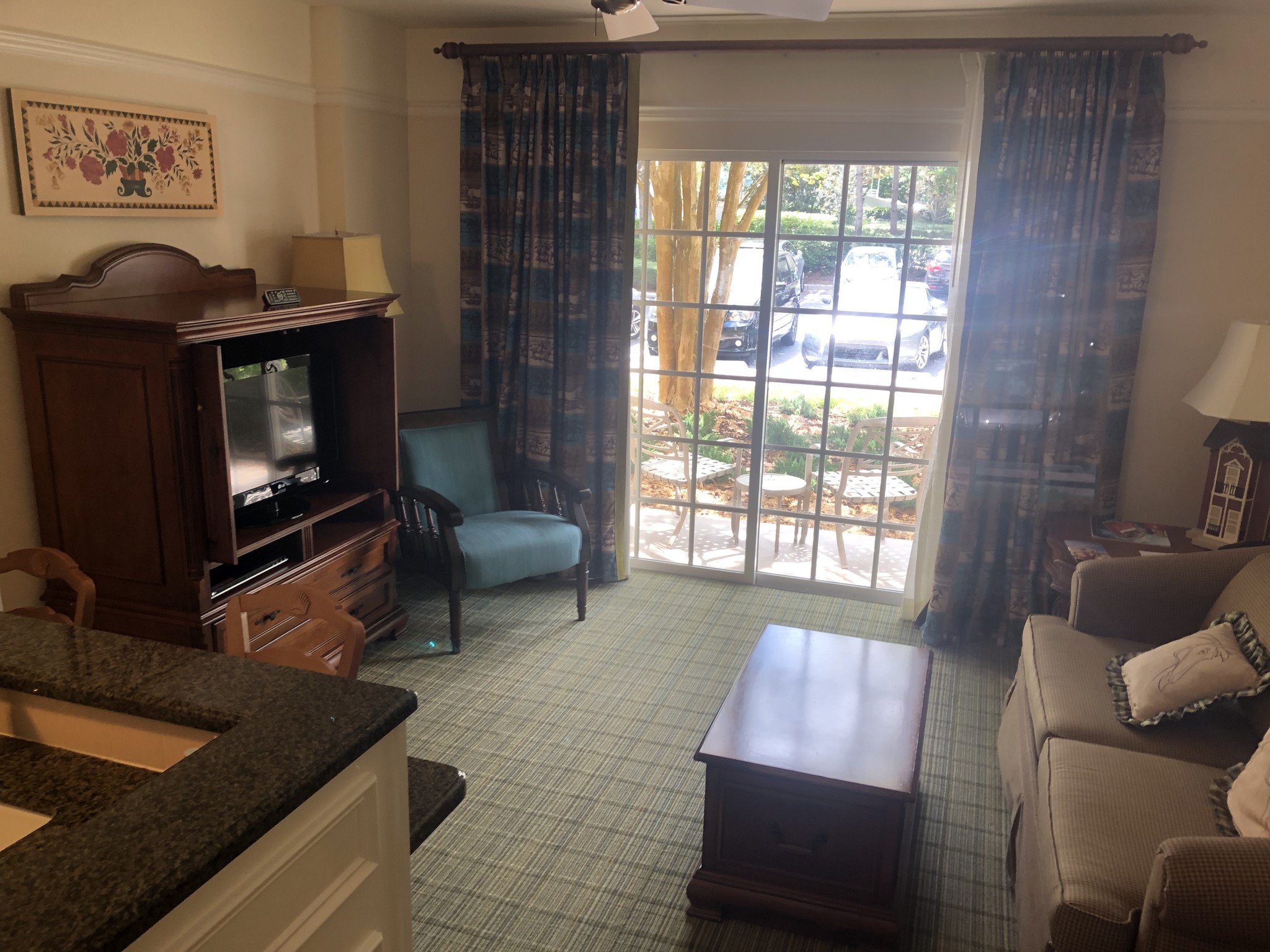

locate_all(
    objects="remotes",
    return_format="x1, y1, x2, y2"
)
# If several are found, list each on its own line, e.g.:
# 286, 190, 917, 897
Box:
264, 287, 301, 305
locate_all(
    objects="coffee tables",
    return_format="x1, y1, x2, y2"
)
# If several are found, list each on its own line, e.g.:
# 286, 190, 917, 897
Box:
685, 625, 936, 952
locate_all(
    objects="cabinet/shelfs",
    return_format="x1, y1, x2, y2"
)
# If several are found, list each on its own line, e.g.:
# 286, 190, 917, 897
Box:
0, 243, 409, 660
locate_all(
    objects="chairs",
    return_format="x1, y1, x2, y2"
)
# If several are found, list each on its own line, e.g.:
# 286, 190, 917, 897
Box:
394, 401, 594, 652
225, 583, 367, 679
0, 547, 97, 630
800, 415, 939, 569
629, 395, 746, 548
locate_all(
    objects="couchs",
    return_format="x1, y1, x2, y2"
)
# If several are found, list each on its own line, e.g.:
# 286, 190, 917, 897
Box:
994, 545, 1270, 952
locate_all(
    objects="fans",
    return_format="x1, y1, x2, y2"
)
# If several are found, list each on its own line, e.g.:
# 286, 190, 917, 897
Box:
600, 0, 834, 41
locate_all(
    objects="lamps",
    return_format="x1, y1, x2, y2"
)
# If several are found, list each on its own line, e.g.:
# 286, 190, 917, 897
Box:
591, 0, 640, 37
288, 224, 404, 319
1180, 320, 1270, 550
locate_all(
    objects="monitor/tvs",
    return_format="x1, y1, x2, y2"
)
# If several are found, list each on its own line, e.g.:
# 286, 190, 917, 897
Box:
217, 347, 346, 528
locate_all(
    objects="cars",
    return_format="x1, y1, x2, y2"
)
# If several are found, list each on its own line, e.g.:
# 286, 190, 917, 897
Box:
756, 239, 807, 292
801, 280, 949, 370
630, 288, 642, 340
925, 249, 951, 295
833, 247, 904, 288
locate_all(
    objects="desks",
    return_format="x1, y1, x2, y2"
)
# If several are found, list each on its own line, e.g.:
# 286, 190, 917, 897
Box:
1034, 507, 1211, 622
737, 472, 807, 560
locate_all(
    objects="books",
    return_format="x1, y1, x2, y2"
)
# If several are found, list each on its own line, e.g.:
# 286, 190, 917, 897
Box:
1064, 540, 1107, 564
1090, 516, 1171, 548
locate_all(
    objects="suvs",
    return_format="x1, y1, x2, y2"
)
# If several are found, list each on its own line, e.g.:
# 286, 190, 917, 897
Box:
647, 244, 801, 362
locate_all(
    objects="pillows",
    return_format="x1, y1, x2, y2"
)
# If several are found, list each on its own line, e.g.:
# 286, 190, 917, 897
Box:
1104, 610, 1270, 731
1207, 727, 1270, 838
